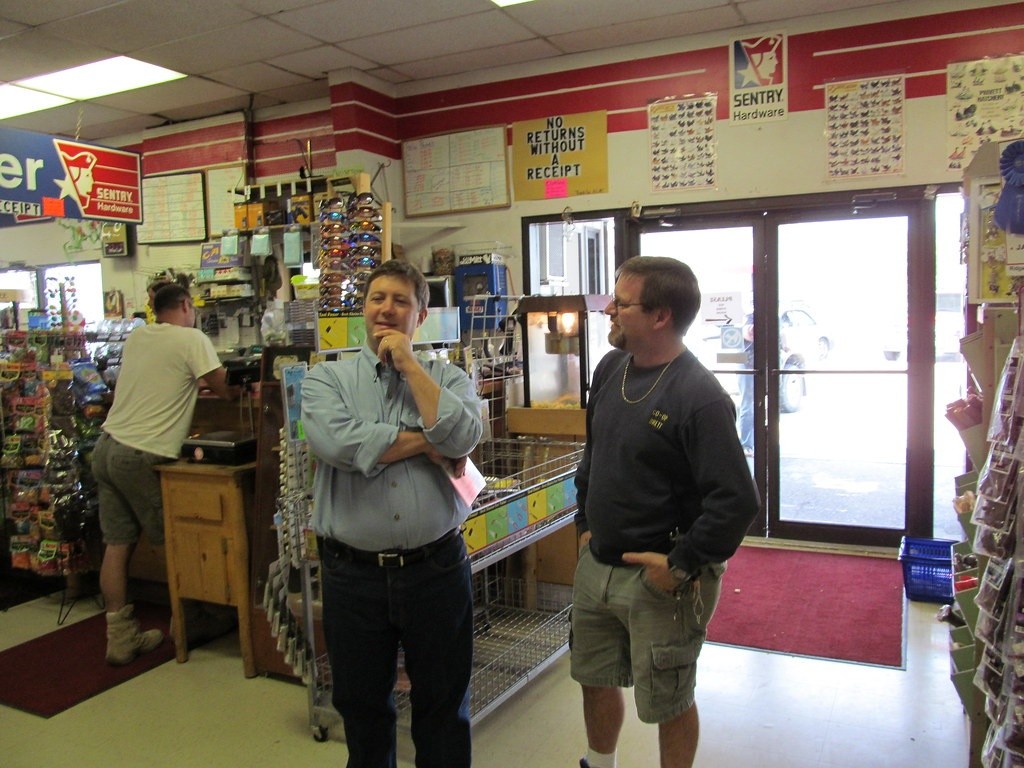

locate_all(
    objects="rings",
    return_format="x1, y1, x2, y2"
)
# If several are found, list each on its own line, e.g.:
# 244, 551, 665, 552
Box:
385, 339, 387, 343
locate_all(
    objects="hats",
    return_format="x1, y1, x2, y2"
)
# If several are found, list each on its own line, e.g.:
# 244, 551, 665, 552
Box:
262, 256, 282, 291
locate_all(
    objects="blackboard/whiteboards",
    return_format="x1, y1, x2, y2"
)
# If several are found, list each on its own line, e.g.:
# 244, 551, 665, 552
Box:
136, 173, 209, 246
400, 124, 512, 219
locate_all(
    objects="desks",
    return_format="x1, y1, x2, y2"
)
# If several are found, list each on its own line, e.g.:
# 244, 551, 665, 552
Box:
158, 457, 259, 678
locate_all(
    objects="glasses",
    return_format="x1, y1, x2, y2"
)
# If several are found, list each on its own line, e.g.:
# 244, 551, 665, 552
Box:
314, 191, 384, 314
610, 292, 662, 309
190, 301, 201, 316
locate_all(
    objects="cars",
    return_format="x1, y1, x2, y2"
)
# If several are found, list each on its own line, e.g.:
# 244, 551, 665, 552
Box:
737, 307, 834, 414
880, 301, 962, 361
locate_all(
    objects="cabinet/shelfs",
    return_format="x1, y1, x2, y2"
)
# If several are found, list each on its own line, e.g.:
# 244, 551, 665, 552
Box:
0, 324, 163, 624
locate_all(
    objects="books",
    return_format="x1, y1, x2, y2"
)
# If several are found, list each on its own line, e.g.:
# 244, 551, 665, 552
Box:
439, 455, 485, 508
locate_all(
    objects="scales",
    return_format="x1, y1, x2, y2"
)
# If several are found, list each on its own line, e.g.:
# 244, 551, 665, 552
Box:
179, 359, 264, 467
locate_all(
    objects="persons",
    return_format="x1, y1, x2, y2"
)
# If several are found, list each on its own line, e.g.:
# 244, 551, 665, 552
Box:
568, 255, 761, 768
146, 282, 171, 314
737, 310, 791, 457
86, 284, 247, 662
301, 259, 483, 768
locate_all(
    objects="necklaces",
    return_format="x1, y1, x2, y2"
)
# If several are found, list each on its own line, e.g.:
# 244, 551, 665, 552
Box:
621, 345, 688, 404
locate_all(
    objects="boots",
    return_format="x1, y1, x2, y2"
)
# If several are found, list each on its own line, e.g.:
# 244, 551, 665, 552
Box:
105, 604, 164, 664
169, 599, 238, 642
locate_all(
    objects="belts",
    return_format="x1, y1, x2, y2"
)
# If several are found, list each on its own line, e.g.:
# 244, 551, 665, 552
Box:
316, 526, 461, 569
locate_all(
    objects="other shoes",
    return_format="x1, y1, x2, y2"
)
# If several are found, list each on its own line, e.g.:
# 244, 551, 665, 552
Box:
580, 758, 590, 768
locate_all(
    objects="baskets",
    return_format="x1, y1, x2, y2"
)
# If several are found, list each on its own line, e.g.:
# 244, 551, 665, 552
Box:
898, 536, 961, 605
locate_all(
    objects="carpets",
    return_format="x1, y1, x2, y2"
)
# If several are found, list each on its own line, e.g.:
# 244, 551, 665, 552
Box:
705, 545, 909, 670
